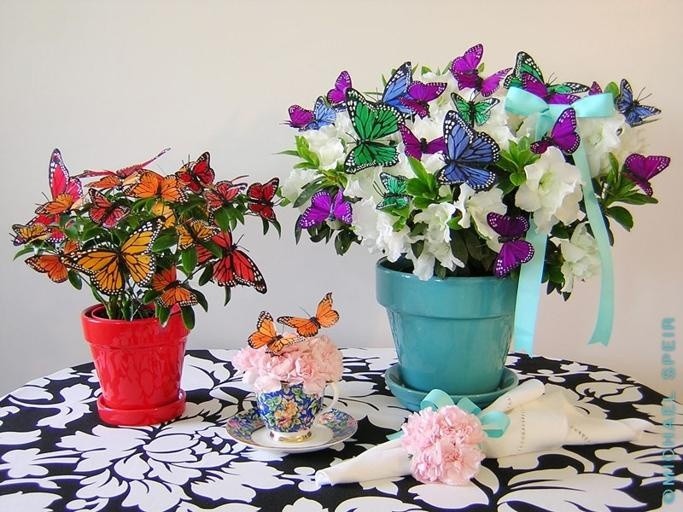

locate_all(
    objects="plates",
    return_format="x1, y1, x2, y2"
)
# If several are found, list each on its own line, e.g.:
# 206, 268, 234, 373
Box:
225, 406, 358, 454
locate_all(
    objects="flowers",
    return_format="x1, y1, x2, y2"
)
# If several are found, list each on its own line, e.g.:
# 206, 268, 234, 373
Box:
401, 406, 487, 487
233, 335, 343, 398
9, 150, 280, 331
274, 42, 670, 302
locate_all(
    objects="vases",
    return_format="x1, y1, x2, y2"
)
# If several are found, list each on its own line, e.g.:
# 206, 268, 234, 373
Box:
82, 303, 189, 426
375, 255, 520, 413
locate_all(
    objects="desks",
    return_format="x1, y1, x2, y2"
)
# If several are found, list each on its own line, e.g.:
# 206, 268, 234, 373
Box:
0, 350, 683, 512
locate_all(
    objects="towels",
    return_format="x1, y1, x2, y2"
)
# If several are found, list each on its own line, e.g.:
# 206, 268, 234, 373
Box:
314, 379, 608, 486
